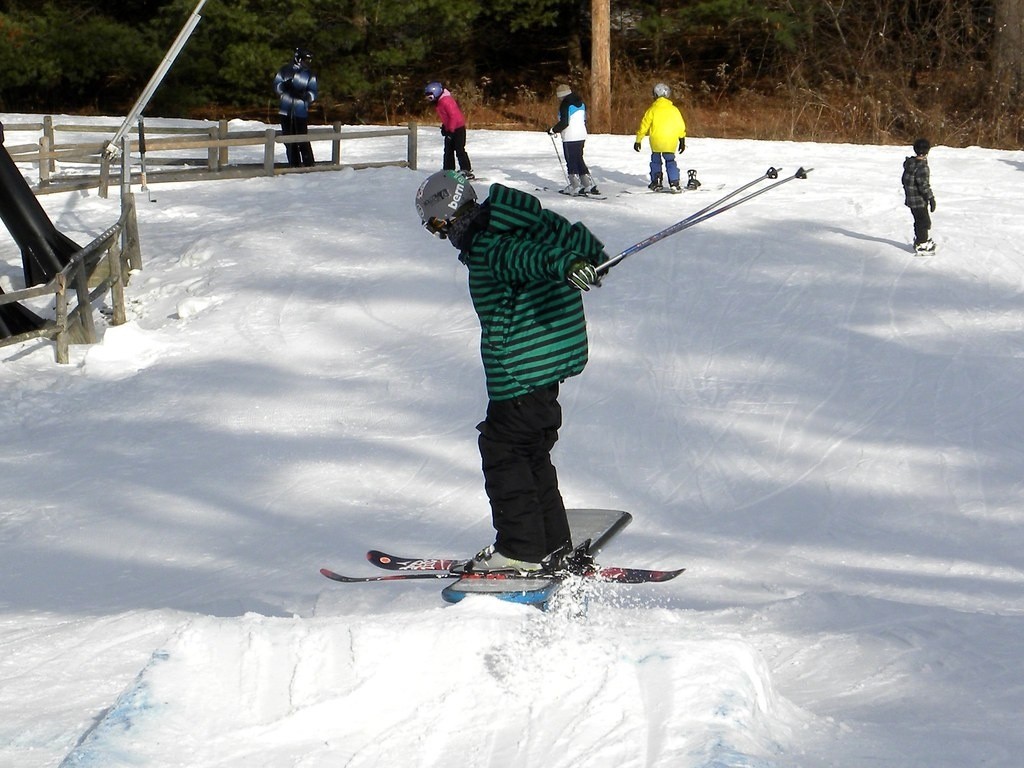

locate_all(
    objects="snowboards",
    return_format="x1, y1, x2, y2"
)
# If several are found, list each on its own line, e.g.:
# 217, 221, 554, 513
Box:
913, 246, 937, 258
419, 169, 490, 182
621, 183, 726, 194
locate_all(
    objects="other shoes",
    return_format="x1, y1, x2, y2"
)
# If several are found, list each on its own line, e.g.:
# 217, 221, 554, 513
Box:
562, 185, 579, 196
670, 182, 680, 190
648, 181, 663, 190
579, 187, 599, 195
914, 241, 935, 252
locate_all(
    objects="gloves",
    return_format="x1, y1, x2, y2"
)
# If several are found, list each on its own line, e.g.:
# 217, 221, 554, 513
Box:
289, 90, 309, 102
282, 80, 294, 93
566, 253, 610, 291
545, 127, 555, 136
444, 133, 453, 145
929, 197, 936, 212
440, 125, 446, 136
634, 142, 641, 152
678, 138, 685, 155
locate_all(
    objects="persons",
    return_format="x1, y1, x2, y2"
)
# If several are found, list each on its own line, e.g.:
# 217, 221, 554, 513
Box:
901, 138, 936, 251
273, 47, 318, 162
547, 84, 599, 195
425, 82, 475, 180
634, 83, 687, 190
415, 170, 610, 570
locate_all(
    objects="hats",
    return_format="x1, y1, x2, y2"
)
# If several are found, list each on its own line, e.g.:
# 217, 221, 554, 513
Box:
556, 84, 572, 97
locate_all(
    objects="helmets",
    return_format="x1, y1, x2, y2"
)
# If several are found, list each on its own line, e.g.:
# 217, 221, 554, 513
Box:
913, 139, 931, 155
294, 47, 315, 71
654, 83, 670, 99
424, 82, 443, 98
415, 170, 478, 239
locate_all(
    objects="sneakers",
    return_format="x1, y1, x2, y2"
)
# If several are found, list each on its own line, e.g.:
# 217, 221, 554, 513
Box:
546, 545, 574, 568
469, 546, 554, 573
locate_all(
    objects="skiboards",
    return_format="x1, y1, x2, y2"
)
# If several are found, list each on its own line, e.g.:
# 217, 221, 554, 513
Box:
535, 187, 607, 201
319, 549, 685, 584
